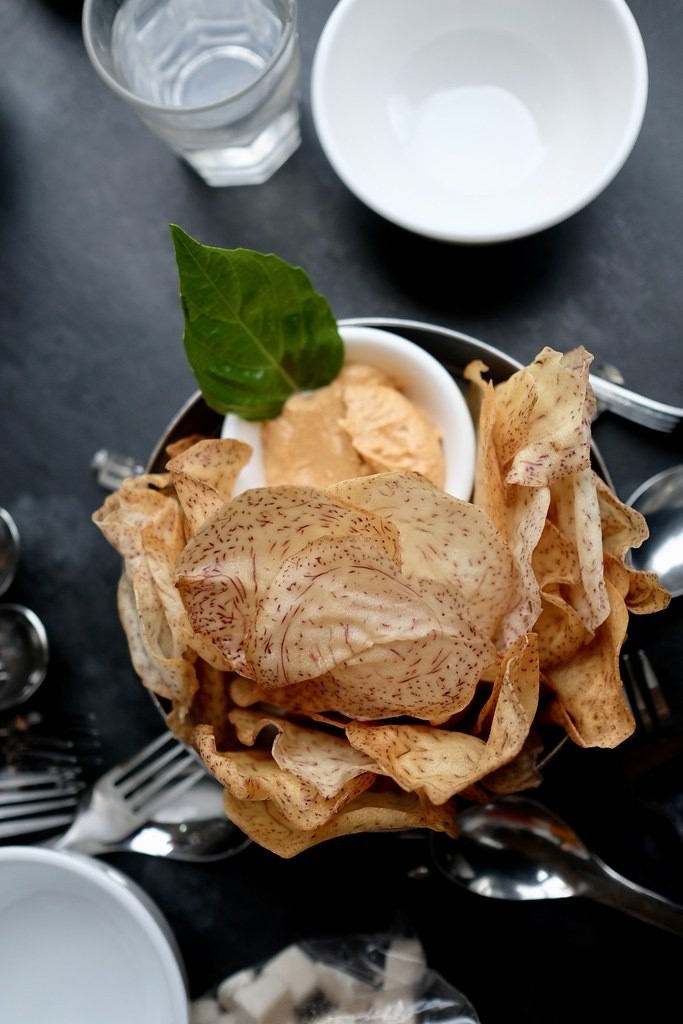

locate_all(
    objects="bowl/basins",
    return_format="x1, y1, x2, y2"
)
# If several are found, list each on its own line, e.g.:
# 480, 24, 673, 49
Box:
311, 0, 647, 240
145, 319, 617, 817
0, 847, 190, 1024
221, 326, 475, 508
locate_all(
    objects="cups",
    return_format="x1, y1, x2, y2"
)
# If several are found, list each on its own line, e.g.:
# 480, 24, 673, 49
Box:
84, 0, 302, 188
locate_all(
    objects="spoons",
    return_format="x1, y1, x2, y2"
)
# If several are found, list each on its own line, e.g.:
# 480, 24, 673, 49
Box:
127, 781, 253, 860
460, 816, 683, 933
0, 601, 50, 712
625, 467, 683, 597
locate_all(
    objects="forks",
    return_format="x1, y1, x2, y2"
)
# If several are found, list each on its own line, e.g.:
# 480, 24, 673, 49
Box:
0, 770, 76, 837
55, 731, 206, 856
609, 650, 683, 774
